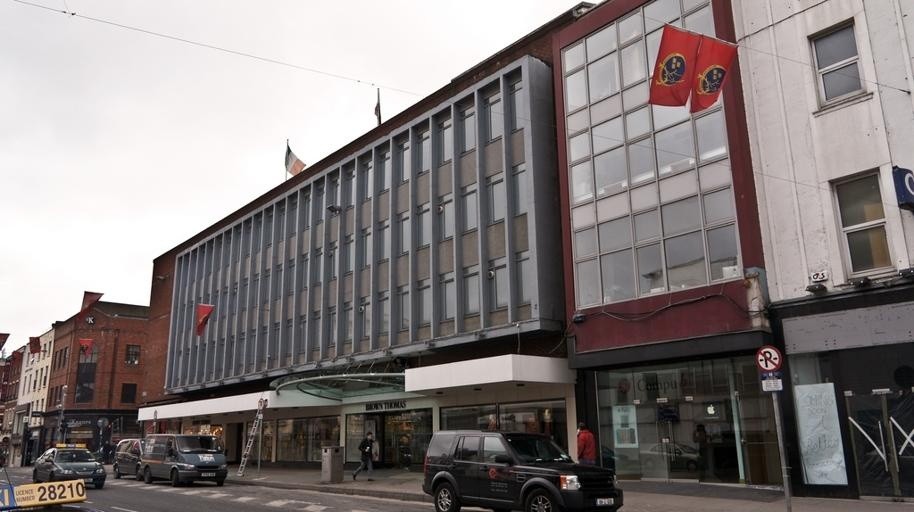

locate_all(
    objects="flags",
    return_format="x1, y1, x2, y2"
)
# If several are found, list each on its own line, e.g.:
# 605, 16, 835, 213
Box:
689, 35, 737, 114
79, 338, 94, 358
648, 23, 703, 110
195, 303, 214, 335
286, 146, 305, 176
374, 99, 380, 121
0, 334, 10, 350
29, 337, 41, 354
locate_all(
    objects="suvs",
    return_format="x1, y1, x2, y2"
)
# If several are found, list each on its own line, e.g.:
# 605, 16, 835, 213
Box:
421, 429, 622, 512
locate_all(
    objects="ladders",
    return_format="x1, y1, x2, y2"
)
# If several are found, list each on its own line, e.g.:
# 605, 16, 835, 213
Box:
236, 409, 261, 476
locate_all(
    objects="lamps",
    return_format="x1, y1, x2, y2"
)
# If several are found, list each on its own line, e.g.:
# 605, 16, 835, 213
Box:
848, 276, 869, 286
898, 268, 913, 278
807, 283, 826, 293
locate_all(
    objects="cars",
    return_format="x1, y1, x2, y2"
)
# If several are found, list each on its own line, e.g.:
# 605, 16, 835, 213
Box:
91, 446, 114, 463
33, 442, 104, 490
113, 438, 143, 481
640, 442, 702, 475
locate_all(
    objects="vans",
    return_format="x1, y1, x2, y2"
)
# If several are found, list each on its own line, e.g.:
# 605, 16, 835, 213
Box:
139, 432, 228, 487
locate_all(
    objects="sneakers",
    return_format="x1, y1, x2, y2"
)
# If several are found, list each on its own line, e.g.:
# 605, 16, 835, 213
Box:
368, 478, 374, 482
352, 473, 356, 481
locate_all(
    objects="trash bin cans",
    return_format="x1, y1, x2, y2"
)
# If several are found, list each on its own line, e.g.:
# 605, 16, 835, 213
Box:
321, 446, 344, 484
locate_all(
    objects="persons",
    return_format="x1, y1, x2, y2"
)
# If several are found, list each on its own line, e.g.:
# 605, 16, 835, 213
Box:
398, 435, 412, 470
542, 407, 552, 437
216, 442, 224, 455
488, 414, 496, 431
0, 448, 9, 473
351, 432, 375, 481
88, 439, 120, 465
693, 423, 725, 481
577, 422, 597, 465
25, 448, 43, 465
